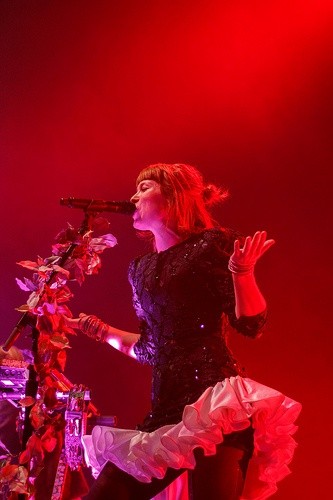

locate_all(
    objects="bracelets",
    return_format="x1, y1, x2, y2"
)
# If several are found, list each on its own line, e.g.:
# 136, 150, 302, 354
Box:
227, 258, 254, 274
77, 315, 110, 344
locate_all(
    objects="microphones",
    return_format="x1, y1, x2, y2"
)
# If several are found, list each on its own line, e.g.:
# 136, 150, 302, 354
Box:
60, 198, 136, 216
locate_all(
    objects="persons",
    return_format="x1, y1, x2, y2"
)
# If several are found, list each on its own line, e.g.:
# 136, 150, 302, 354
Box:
63, 164, 302, 500
